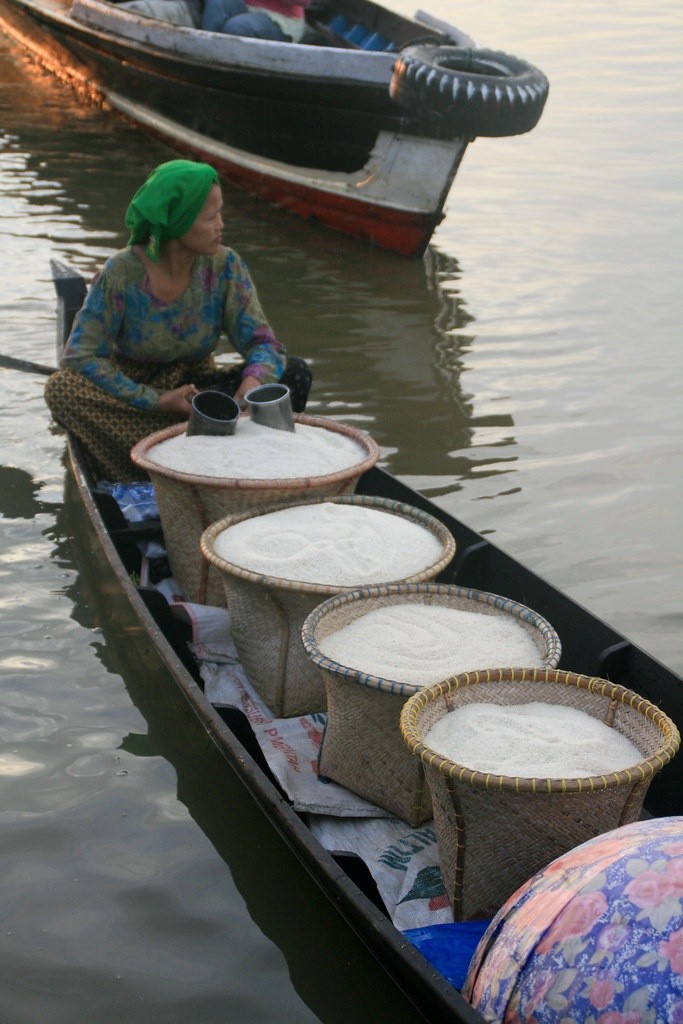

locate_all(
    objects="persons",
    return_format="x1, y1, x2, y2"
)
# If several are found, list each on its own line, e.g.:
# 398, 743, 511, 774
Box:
197, 0, 309, 43
44, 159, 312, 485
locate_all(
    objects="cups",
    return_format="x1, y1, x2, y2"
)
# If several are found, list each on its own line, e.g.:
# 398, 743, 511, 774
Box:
244, 383, 296, 433
185, 390, 240, 436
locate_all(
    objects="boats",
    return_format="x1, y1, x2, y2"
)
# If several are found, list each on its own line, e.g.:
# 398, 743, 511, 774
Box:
45, 256, 683, 1024
2, 0, 551, 265
